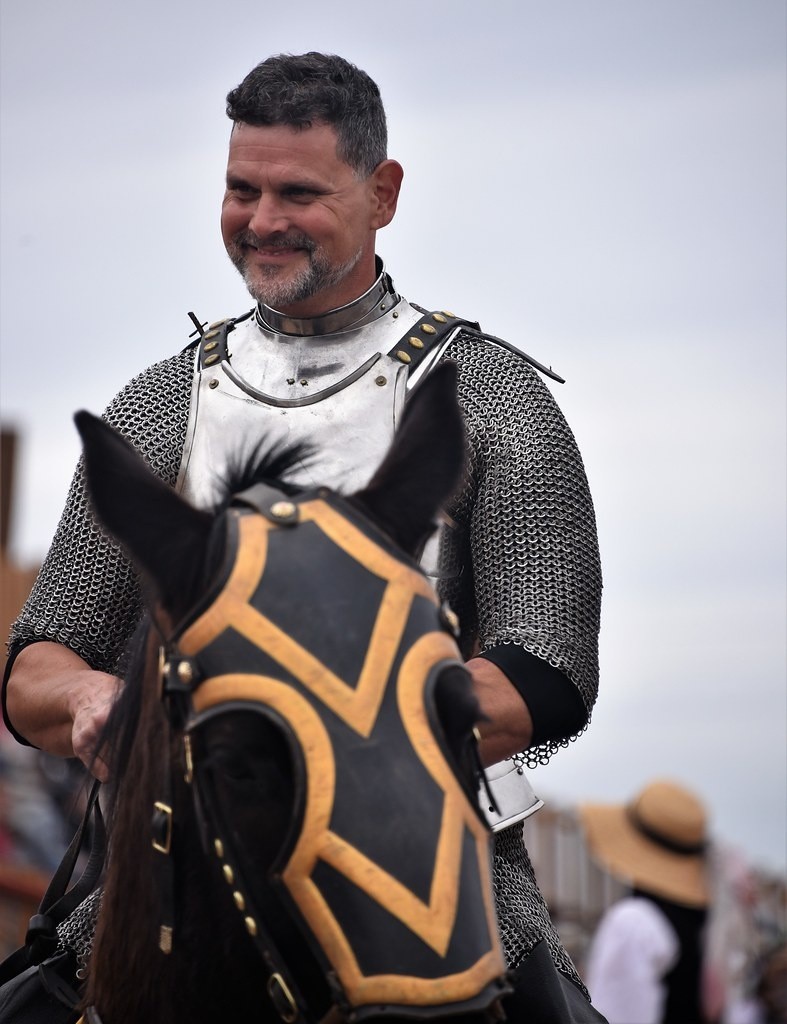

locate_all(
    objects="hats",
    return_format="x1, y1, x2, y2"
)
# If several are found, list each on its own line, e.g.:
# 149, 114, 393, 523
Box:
579, 781, 716, 905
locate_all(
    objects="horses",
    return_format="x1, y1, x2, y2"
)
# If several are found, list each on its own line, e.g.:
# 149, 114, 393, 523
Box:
0, 357, 607, 1023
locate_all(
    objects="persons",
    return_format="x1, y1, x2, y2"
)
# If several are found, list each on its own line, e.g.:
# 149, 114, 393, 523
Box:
0, 51, 603, 1024
572, 780, 787, 1024
